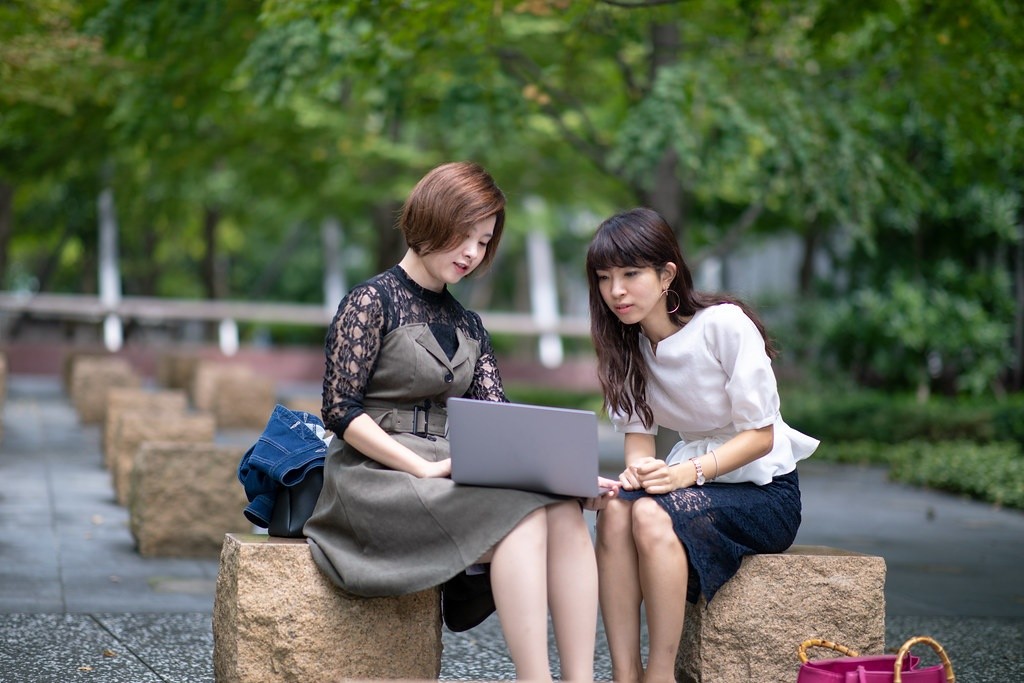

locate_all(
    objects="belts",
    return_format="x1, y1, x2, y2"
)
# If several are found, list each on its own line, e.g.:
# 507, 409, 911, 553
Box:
359, 406, 447, 443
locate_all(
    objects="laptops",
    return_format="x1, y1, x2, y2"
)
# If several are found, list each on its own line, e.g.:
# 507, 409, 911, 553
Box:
447, 397, 612, 498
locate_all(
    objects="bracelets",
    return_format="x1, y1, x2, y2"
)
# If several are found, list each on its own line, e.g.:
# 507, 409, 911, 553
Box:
709, 450, 719, 480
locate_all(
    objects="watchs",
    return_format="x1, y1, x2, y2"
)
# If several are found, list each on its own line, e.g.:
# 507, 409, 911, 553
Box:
692, 458, 706, 486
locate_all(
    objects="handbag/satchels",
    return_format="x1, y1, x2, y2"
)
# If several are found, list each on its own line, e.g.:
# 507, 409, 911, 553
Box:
267, 466, 325, 539
797, 636, 955, 683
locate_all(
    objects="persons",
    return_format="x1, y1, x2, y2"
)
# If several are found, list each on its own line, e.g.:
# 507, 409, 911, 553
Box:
586, 208, 820, 683
303, 162, 623, 683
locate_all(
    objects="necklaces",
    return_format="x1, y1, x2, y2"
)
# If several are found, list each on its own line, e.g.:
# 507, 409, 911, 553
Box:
651, 343, 657, 348
